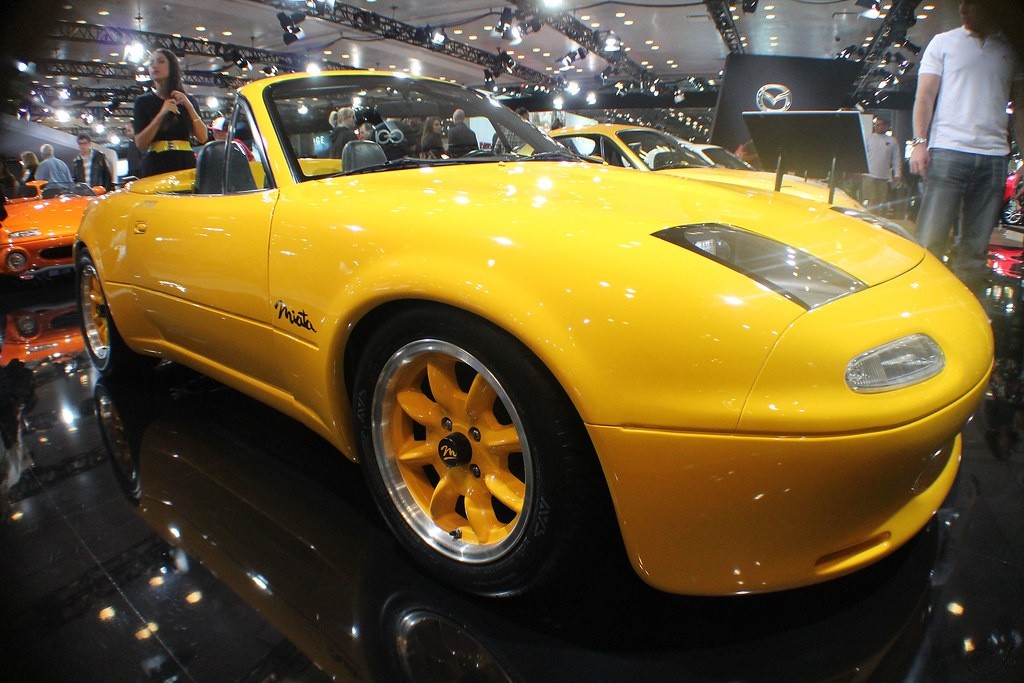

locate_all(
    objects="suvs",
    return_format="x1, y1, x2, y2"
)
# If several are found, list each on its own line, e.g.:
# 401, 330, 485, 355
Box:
844, 148, 920, 209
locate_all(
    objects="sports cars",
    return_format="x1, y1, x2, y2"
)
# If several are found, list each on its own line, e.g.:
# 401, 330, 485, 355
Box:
511, 122, 866, 210
0, 179, 107, 282
74, 67, 997, 599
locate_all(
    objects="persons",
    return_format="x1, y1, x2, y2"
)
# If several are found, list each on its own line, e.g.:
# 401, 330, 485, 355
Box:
909, 0, 1024, 303
207, 117, 256, 162
741, 138, 763, 171
133, 48, 207, 178
72, 134, 113, 190
34, 143, 72, 188
328, 106, 580, 164
0, 150, 39, 223
126, 119, 141, 179
841, 114, 923, 223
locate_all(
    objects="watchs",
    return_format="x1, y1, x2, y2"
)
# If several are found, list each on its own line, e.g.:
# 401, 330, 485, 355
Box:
912, 136, 927, 145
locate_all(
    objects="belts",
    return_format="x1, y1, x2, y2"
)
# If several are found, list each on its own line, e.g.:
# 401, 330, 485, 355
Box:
147, 140, 193, 153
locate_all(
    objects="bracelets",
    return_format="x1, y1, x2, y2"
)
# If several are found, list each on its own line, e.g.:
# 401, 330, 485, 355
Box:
191, 115, 201, 121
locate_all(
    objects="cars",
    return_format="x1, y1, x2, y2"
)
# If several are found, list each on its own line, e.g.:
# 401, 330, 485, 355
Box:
1002, 157, 1024, 225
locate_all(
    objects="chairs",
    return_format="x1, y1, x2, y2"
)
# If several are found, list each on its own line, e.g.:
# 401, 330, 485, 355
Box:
342, 141, 388, 172
192, 140, 258, 194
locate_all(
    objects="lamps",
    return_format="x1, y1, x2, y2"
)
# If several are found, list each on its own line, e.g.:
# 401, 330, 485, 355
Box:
6, 0, 932, 136
